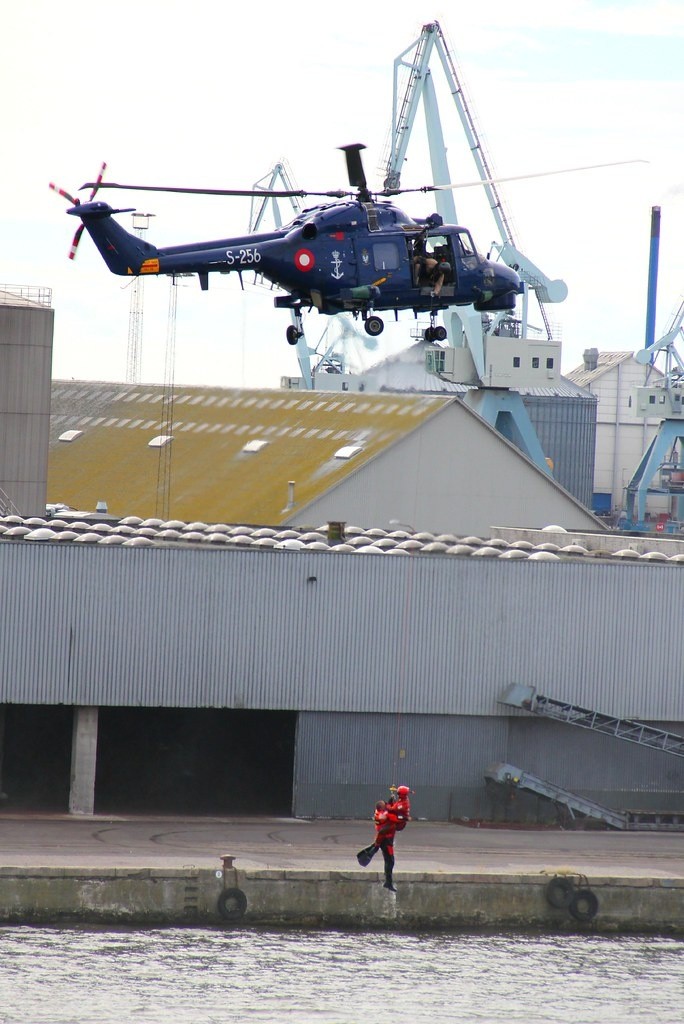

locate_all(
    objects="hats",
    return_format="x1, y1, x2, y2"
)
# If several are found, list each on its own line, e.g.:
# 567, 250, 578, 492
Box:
397, 786, 409, 795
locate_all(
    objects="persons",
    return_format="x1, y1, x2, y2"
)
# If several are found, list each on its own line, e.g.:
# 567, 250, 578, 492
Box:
371, 786, 411, 849
372, 800, 399, 894
411, 256, 451, 298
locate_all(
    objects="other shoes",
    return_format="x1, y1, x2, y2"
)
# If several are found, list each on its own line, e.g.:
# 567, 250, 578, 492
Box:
382, 882, 397, 892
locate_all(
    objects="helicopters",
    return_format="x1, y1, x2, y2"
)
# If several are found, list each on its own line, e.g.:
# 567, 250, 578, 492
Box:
48, 143, 650, 344
